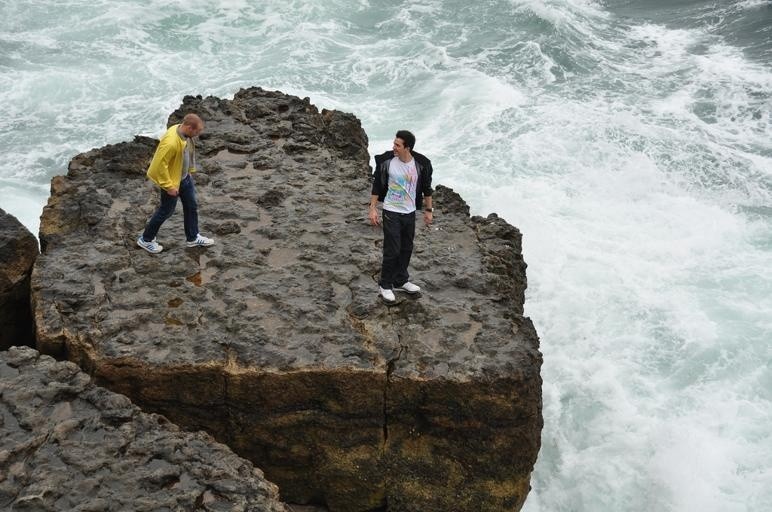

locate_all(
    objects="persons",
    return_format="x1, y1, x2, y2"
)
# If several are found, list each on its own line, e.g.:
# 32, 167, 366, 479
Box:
134, 113, 215, 254
368, 129, 434, 304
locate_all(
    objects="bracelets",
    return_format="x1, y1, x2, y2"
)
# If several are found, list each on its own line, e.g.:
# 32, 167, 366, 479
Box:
425, 207, 434, 213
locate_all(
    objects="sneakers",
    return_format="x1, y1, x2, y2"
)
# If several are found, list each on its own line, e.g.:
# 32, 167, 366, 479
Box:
137, 235, 164, 254
394, 282, 420, 295
186, 234, 215, 248
379, 286, 396, 305
